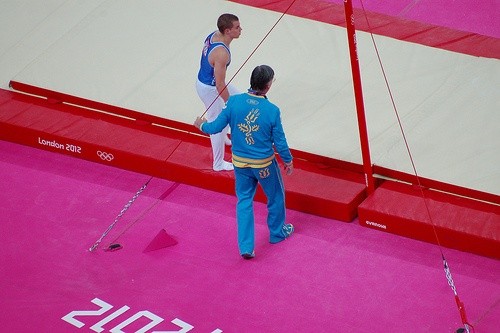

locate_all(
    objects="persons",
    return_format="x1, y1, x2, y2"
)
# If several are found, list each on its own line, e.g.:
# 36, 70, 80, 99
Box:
194, 64, 295, 258
194, 13, 242, 172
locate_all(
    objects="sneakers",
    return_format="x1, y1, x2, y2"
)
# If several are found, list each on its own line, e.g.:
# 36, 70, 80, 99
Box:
281, 223, 294, 238
242, 251, 255, 258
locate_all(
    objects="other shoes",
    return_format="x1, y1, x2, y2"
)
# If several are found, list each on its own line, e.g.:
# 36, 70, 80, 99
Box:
224, 136, 231, 146
215, 162, 237, 171
